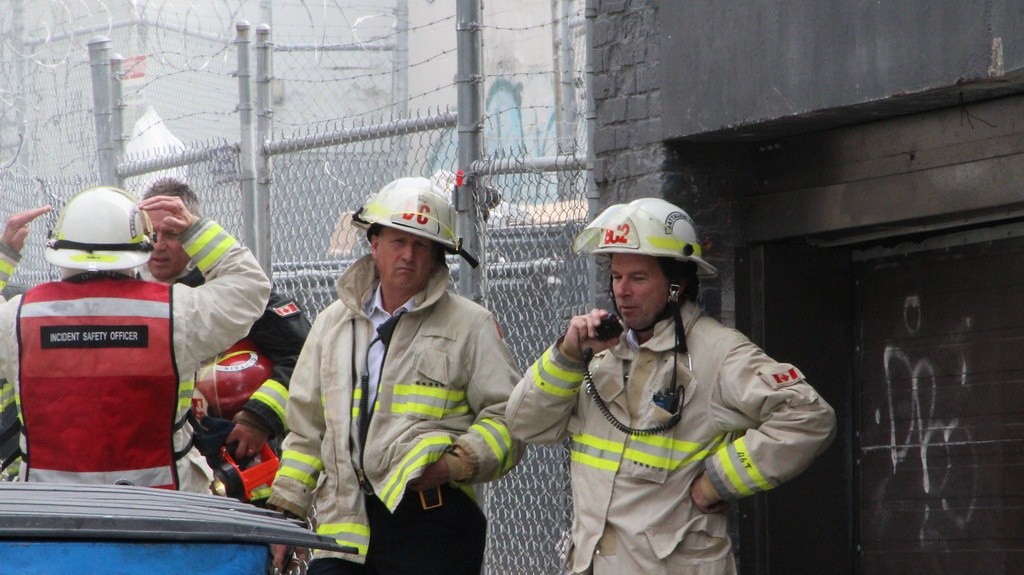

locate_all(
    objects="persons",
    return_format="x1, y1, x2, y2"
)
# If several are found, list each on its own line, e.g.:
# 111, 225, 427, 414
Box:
265, 176, 528, 575
0, 187, 272, 499
505, 197, 837, 575
140, 176, 314, 507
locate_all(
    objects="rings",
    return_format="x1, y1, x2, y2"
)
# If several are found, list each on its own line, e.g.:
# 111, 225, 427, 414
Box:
247, 449, 256, 453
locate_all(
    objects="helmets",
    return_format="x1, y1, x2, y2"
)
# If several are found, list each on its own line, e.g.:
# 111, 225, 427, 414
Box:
351, 177, 480, 269
193, 336, 273, 420
44, 186, 155, 270
591, 198, 720, 278
